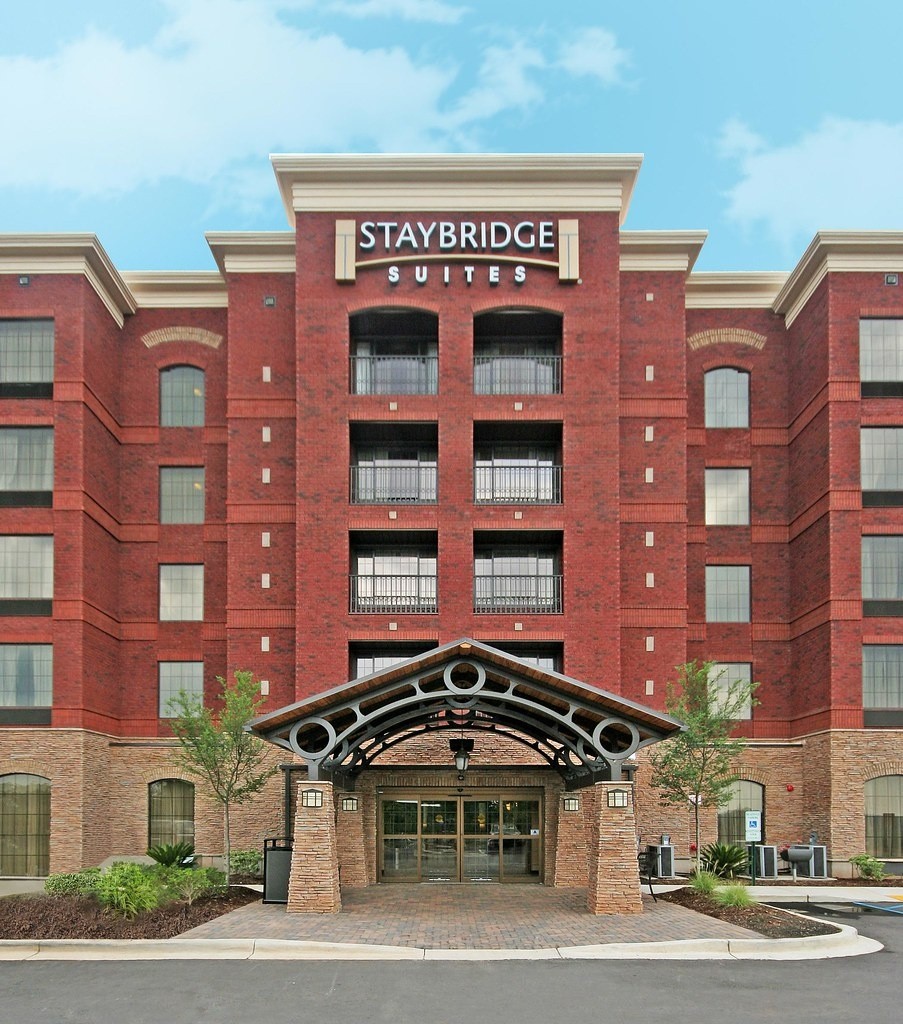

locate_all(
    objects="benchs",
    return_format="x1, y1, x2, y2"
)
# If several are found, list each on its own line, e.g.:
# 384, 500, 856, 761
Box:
638, 852, 661, 902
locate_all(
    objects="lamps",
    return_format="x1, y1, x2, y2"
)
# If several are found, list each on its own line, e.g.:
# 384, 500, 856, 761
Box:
19, 275, 30, 286
607, 789, 628, 808
342, 796, 358, 811
564, 797, 580, 811
302, 788, 323, 808
453, 747, 471, 781
886, 274, 897, 284
265, 296, 275, 306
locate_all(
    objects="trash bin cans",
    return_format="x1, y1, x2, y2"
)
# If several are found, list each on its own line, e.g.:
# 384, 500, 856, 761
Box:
264, 837, 291, 904
